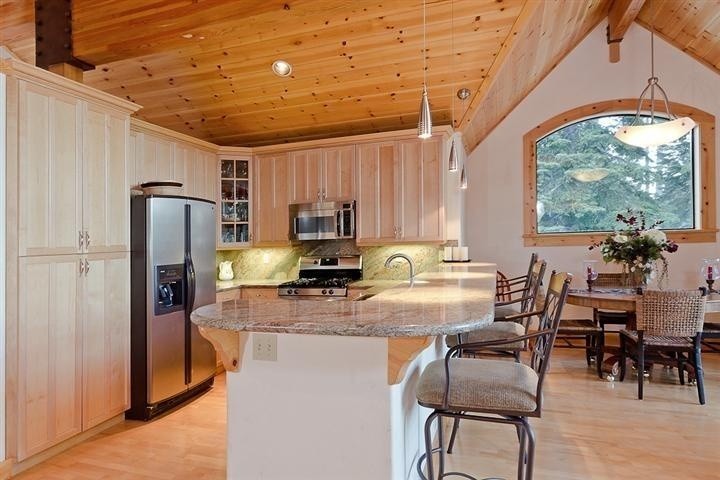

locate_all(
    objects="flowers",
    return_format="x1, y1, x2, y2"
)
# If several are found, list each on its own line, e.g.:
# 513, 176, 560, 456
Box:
587, 209, 680, 290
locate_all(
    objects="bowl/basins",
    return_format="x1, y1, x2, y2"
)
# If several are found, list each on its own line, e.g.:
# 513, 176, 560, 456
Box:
140, 181, 182, 196
131, 189, 144, 196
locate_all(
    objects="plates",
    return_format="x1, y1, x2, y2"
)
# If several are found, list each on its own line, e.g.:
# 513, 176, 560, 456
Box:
442, 260, 471, 263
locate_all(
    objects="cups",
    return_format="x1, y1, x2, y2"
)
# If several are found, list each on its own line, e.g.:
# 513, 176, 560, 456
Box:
443, 246, 469, 261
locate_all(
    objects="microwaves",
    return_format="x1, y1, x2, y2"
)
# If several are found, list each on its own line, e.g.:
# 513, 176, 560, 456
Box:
289, 201, 356, 241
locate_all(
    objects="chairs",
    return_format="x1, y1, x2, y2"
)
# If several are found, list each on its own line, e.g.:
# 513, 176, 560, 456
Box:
530, 277, 606, 379
415, 252, 573, 479
616, 288, 708, 405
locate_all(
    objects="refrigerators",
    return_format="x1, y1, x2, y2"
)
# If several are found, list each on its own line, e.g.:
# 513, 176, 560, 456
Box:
128, 194, 217, 421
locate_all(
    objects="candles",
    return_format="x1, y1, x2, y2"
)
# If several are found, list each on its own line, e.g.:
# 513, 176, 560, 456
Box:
707, 266, 713, 279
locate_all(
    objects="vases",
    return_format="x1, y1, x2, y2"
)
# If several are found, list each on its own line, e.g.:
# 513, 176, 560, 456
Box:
626, 259, 647, 295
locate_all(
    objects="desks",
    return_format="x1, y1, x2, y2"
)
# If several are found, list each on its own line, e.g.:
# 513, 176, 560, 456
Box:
565, 288, 720, 387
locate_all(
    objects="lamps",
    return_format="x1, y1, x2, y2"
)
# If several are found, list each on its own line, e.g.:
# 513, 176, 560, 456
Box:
417, 1, 469, 191
615, 23, 697, 148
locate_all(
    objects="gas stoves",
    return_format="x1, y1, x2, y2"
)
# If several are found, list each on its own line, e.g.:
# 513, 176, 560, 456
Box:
279, 278, 355, 288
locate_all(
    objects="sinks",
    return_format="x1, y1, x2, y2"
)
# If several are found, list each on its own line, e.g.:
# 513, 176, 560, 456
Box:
352, 292, 375, 301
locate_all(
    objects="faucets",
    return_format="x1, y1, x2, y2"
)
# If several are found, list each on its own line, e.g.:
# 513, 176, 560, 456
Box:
384, 253, 415, 279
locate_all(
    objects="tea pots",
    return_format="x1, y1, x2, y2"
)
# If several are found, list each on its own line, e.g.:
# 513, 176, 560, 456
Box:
218, 260, 235, 281
